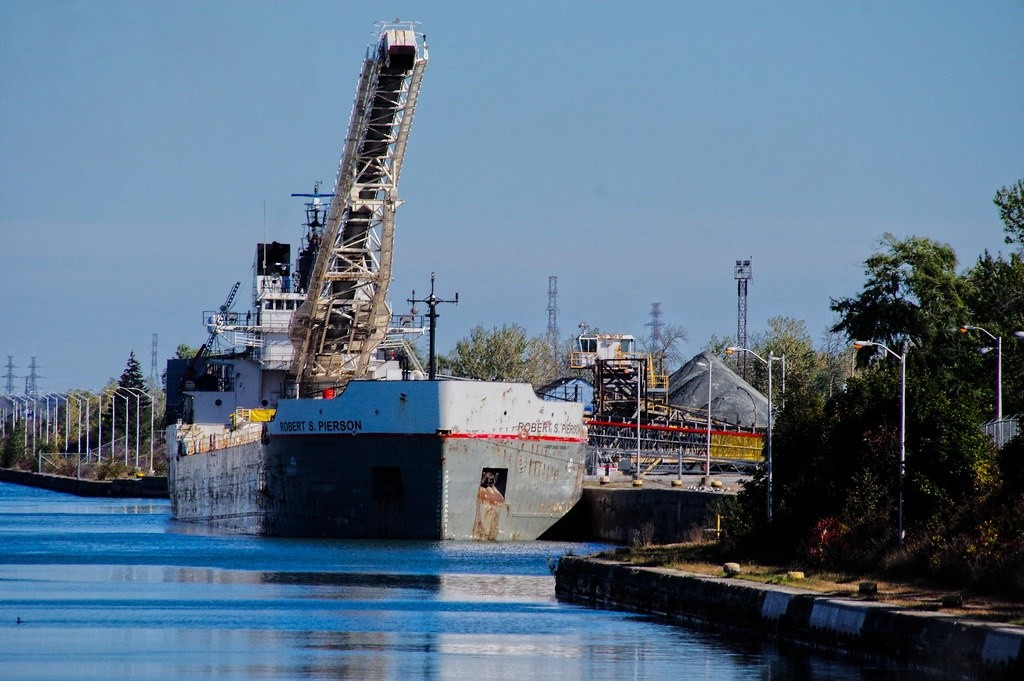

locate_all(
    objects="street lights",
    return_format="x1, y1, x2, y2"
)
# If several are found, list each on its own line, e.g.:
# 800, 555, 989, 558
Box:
960, 325, 1002, 447
105, 390, 128, 466
81, 389, 102, 462
0, 391, 90, 463
725, 347, 772, 522
128, 388, 154, 470
623, 355, 641, 480
117, 387, 140, 468
93, 389, 115, 464
697, 361, 713, 476
854, 340, 906, 540
772, 355, 786, 410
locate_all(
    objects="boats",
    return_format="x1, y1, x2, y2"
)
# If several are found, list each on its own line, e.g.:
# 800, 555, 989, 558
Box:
163, 19, 589, 540
